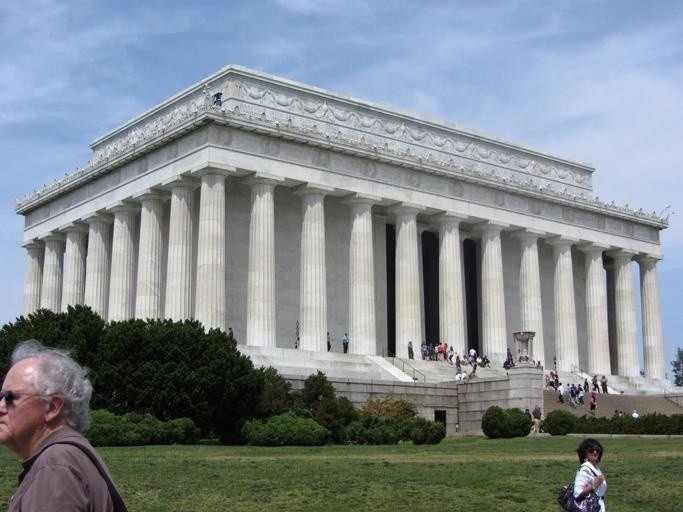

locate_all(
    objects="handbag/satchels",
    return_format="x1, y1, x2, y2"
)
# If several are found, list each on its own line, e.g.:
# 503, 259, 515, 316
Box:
558, 482, 600, 512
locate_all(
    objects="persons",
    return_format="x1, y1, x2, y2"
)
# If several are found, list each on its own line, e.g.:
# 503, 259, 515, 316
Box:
227, 326, 233, 338
525, 405, 541, 434
0, 339, 117, 510
421, 340, 490, 382
327, 332, 331, 351
573, 439, 613, 512
341, 332, 350, 353
504, 348, 514, 371
533, 360, 542, 371
408, 342, 414, 360
615, 409, 639, 417
547, 360, 608, 416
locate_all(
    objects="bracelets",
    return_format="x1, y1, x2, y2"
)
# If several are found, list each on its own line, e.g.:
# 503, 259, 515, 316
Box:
592, 485, 595, 491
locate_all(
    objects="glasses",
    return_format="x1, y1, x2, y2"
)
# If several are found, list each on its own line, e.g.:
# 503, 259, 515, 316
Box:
0, 388, 49, 403
587, 446, 601, 455
